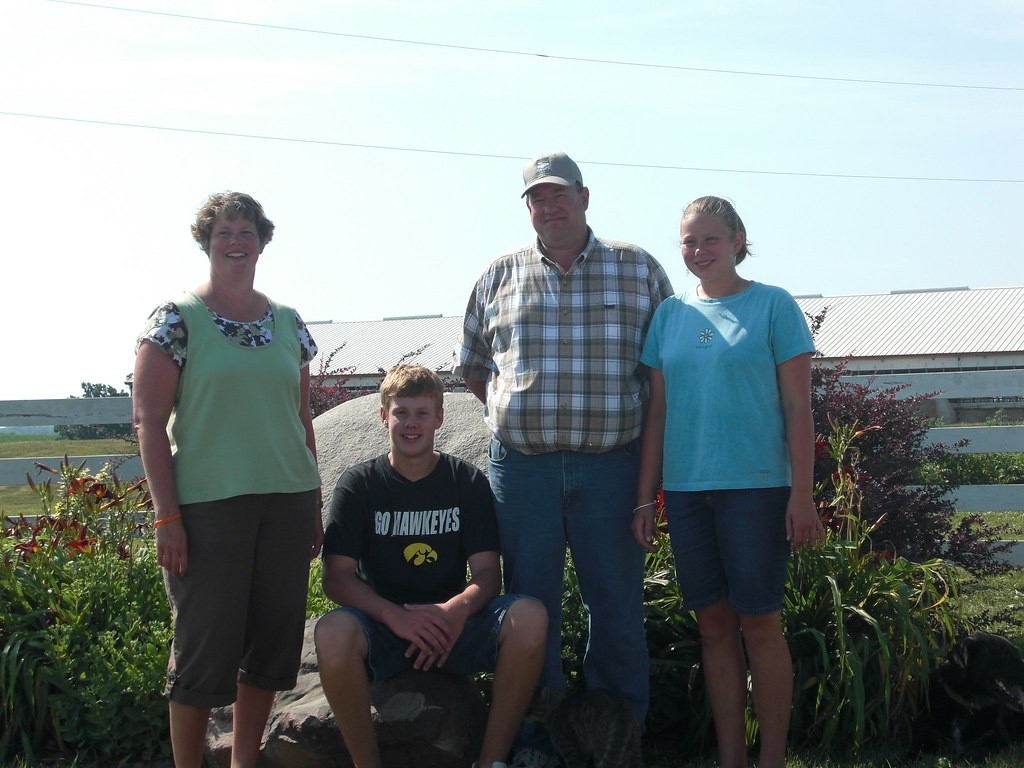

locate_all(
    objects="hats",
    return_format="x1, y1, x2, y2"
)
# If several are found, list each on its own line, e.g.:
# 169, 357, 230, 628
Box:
521, 152, 583, 198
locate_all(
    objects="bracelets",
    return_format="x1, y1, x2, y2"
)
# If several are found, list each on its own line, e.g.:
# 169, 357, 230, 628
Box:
153, 513, 181, 526
632, 502, 656, 514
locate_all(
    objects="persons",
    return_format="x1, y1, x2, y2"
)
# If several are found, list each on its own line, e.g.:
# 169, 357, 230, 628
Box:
314, 364, 548, 767
630, 196, 825, 768
133, 192, 322, 768
450, 151, 674, 768
